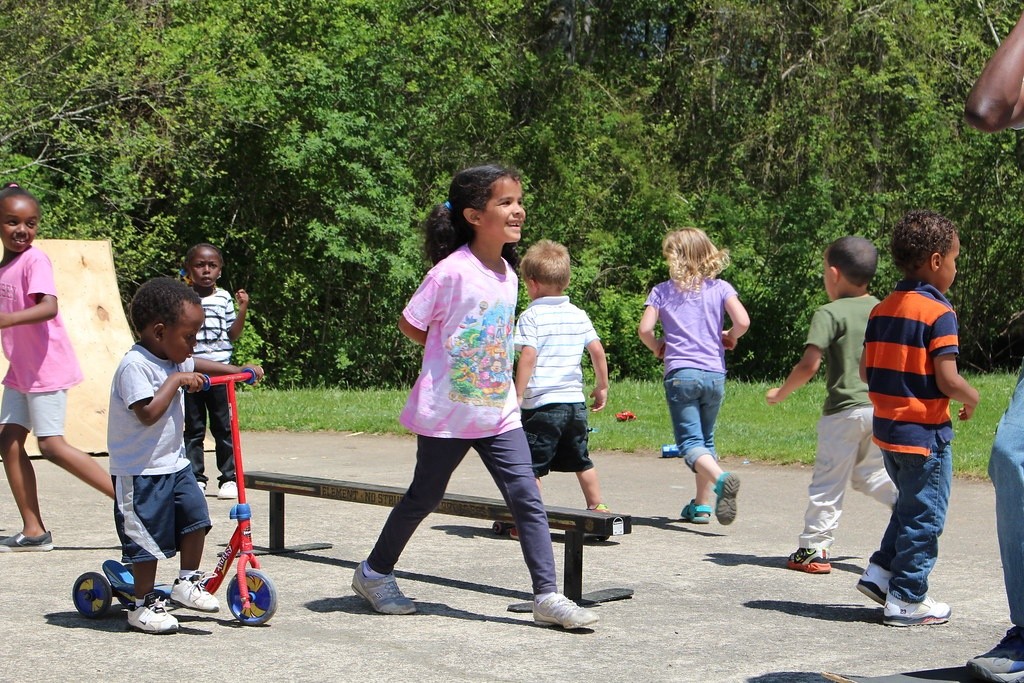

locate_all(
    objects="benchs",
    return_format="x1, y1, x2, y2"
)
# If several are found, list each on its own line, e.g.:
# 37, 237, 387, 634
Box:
217, 469, 634, 615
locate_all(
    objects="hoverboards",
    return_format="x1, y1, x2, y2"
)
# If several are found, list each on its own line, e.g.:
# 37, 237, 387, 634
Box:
72, 365, 278, 628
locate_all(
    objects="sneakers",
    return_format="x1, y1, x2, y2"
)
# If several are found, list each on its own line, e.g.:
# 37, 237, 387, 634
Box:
534, 594, 600, 630
352, 560, 416, 613
511, 528, 520, 540
967, 629, 1024, 683
170, 571, 221, 613
788, 548, 831, 573
0, 532, 53, 552
587, 504, 607, 512
218, 481, 238, 498
855, 563, 894, 605
128, 592, 178, 634
197, 482, 206, 495
883, 589, 951, 626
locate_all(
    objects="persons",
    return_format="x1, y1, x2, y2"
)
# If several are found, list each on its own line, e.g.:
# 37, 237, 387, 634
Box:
768, 235, 899, 574
349, 165, 600, 629
106, 277, 220, 635
187, 244, 248, 500
638, 228, 751, 526
860, 209, 978, 629
509, 239, 614, 540
0, 183, 114, 548
965, 9, 1024, 683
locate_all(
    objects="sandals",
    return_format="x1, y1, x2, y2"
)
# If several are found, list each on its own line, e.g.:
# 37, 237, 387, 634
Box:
681, 499, 711, 523
715, 472, 740, 525
